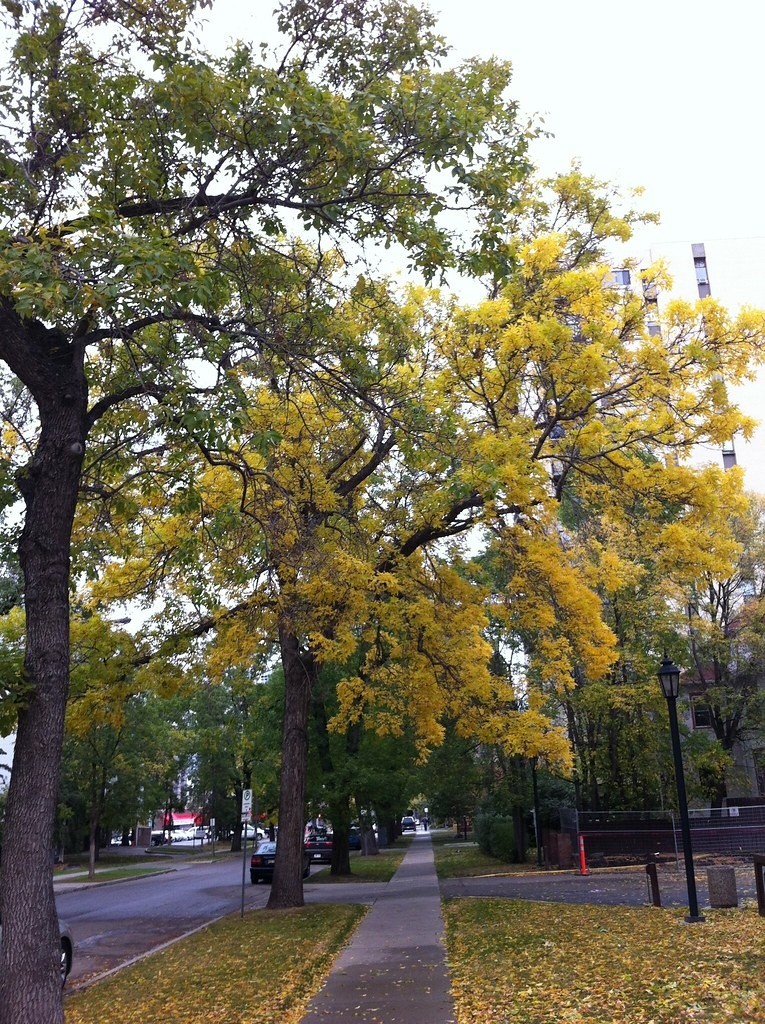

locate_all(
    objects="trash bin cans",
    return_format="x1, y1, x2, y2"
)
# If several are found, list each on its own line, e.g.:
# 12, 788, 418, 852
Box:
706, 865, 739, 908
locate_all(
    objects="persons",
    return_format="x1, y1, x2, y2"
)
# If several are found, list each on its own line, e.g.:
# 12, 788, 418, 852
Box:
421, 816, 428, 831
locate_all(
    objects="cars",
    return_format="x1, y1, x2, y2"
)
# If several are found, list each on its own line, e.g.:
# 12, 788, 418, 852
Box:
348, 826, 362, 850
304, 833, 333, 864
251, 840, 310, 883
401, 817, 416, 832
109, 820, 279, 846
